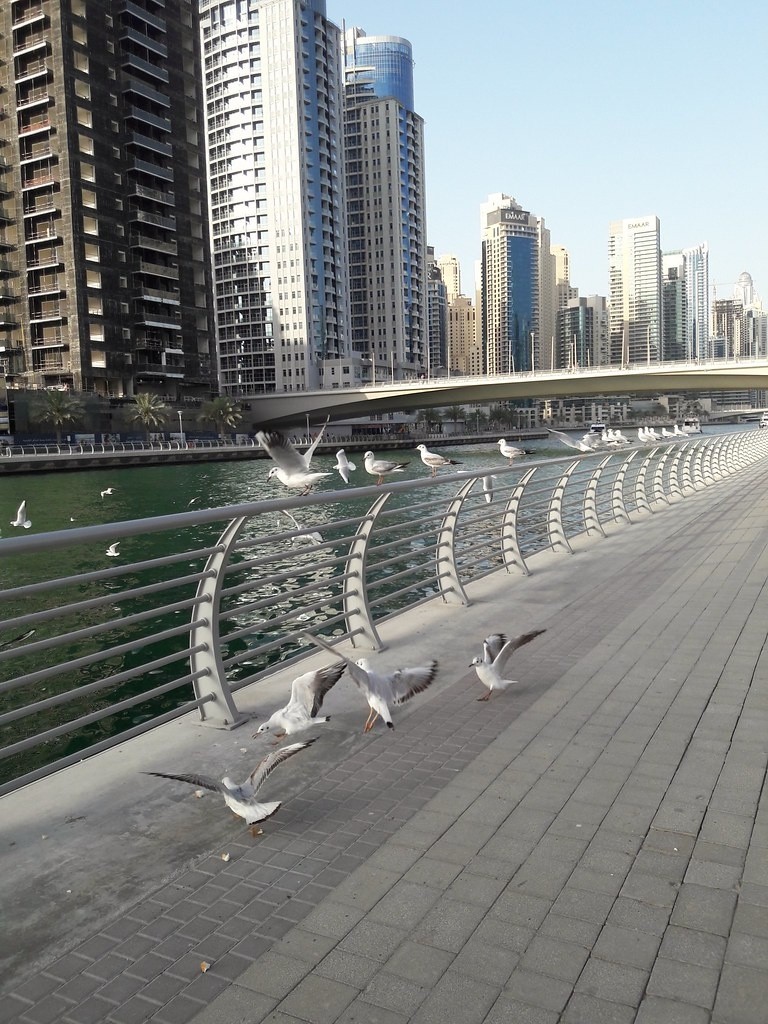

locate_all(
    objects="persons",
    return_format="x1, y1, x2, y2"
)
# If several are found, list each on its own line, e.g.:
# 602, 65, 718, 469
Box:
292, 432, 335, 443
0, 440, 9, 453
66, 432, 120, 449
152, 432, 163, 441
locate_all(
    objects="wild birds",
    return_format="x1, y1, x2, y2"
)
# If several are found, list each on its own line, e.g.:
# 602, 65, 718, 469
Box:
8, 414, 688, 557
251, 658, 347, 739
140, 735, 320, 826
468, 629, 547, 701
304, 631, 438, 732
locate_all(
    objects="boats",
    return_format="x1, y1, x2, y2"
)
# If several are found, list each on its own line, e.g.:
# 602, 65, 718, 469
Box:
589, 424, 606, 435
759, 412, 768, 426
681, 418, 702, 435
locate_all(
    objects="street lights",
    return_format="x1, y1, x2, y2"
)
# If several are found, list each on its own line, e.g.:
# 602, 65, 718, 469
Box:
177, 411, 184, 449
